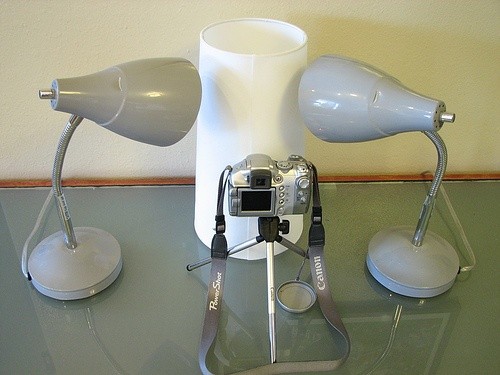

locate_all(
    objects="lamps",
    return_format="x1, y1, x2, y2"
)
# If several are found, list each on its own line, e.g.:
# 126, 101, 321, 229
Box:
299, 51, 478, 298
13, 54, 203, 302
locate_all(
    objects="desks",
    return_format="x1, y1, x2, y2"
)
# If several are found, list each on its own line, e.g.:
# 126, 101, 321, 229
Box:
0, 178, 500, 374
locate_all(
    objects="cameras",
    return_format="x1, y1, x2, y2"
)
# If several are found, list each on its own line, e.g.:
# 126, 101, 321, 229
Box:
229, 154, 312, 217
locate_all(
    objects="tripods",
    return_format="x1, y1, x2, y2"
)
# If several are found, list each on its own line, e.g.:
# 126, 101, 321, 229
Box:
187, 216, 309, 365
